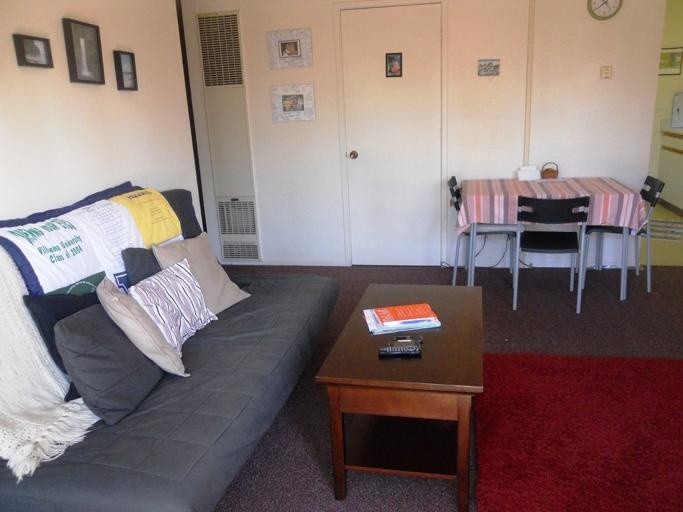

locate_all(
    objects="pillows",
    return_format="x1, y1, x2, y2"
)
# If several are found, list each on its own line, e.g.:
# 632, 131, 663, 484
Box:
125, 258, 220, 358
95, 275, 192, 378
52, 303, 163, 426
22, 291, 102, 404
121, 246, 161, 288
151, 231, 252, 317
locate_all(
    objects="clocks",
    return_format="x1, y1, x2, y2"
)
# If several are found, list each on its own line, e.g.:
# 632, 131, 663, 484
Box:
586, 0, 623, 21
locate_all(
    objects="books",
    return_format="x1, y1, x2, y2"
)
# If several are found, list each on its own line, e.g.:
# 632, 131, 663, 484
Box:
362, 302, 442, 336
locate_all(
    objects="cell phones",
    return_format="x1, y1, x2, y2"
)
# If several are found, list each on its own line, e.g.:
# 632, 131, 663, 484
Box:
393, 335, 424, 343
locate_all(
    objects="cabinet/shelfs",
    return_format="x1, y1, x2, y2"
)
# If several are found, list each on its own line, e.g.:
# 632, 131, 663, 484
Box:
658, 130, 683, 218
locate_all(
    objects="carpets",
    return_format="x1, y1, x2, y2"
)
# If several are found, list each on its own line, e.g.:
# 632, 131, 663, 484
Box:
636, 220, 682, 240
470, 351, 683, 512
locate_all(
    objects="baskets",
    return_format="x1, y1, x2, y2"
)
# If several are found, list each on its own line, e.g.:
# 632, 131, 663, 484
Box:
540, 161, 559, 179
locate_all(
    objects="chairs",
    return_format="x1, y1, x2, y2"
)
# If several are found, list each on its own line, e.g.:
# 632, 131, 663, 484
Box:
581, 174, 665, 303
511, 194, 592, 314
446, 175, 513, 290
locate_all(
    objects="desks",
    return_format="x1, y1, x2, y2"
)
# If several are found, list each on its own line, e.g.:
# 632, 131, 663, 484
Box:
454, 177, 649, 302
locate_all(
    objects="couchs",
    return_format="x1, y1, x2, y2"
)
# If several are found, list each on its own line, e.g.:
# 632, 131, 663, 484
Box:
0, 181, 340, 512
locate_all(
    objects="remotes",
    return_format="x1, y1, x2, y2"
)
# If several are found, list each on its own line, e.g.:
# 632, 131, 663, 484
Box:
379, 345, 422, 357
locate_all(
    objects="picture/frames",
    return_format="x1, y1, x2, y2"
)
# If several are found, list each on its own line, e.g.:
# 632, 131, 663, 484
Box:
385, 52, 402, 77
61, 18, 104, 84
113, 50, 138, 90
657, 47, 682, 75
266, 27, 313, 69
11, 34, 53, 67
270, 83, 316, 121
